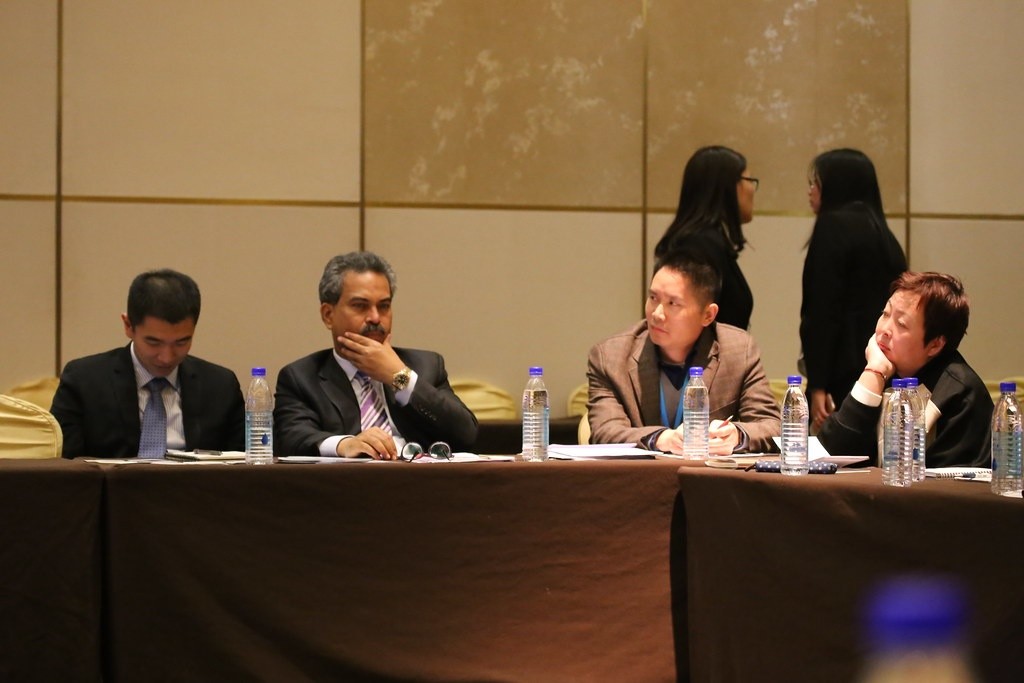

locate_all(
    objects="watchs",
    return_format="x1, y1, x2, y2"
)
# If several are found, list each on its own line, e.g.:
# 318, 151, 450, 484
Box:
650, 428, 667, 451
390, 367, 411, 395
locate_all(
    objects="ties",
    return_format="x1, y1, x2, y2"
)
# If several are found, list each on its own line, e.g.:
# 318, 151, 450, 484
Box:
354, 370, 393, 438
138, 378, 170, 460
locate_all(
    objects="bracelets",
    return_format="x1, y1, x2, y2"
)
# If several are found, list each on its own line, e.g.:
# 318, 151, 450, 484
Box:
864, 369, 887, 385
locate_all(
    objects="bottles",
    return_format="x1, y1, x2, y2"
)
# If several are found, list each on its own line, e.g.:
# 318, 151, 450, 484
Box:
781, 376, 809, 476
245, 368, 274, 466
991, 383, 1024, 495
853, 579, 982, 683
522, 366, 549, 462
883, 379, 912, 486
683, 366, 709, 460
907, 376, 925, 482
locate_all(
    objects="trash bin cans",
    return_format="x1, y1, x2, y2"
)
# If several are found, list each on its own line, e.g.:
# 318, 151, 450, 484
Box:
714, 414, 735, 430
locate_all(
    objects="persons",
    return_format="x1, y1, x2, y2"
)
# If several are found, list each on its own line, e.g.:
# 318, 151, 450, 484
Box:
798, 148, 910, 435
586, 247, 783, 455
653, 145, 759, 331
817, 271, 998, 471
272, 251, 480, 461
49, 268, 245, 458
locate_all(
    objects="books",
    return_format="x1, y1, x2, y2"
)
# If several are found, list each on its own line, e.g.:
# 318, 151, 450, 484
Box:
166, 448, 245, 462
925, 467, 992, 478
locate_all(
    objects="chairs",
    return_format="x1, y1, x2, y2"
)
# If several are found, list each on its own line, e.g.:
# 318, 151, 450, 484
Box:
0, 369, 1024, 458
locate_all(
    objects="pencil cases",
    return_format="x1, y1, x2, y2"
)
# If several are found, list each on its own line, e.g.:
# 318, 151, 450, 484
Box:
744, 460, 837, 475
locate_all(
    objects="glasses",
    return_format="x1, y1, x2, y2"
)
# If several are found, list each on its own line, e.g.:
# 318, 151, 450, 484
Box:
401, 441, 454, 463
739, 176, 758, 191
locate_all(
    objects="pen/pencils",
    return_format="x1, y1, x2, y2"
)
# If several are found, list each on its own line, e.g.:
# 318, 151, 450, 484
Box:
954, 472, 991, 478
193, 448, 223, 456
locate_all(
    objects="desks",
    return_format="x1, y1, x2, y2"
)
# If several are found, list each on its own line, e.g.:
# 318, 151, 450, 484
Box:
0, 447, 1024, 683
